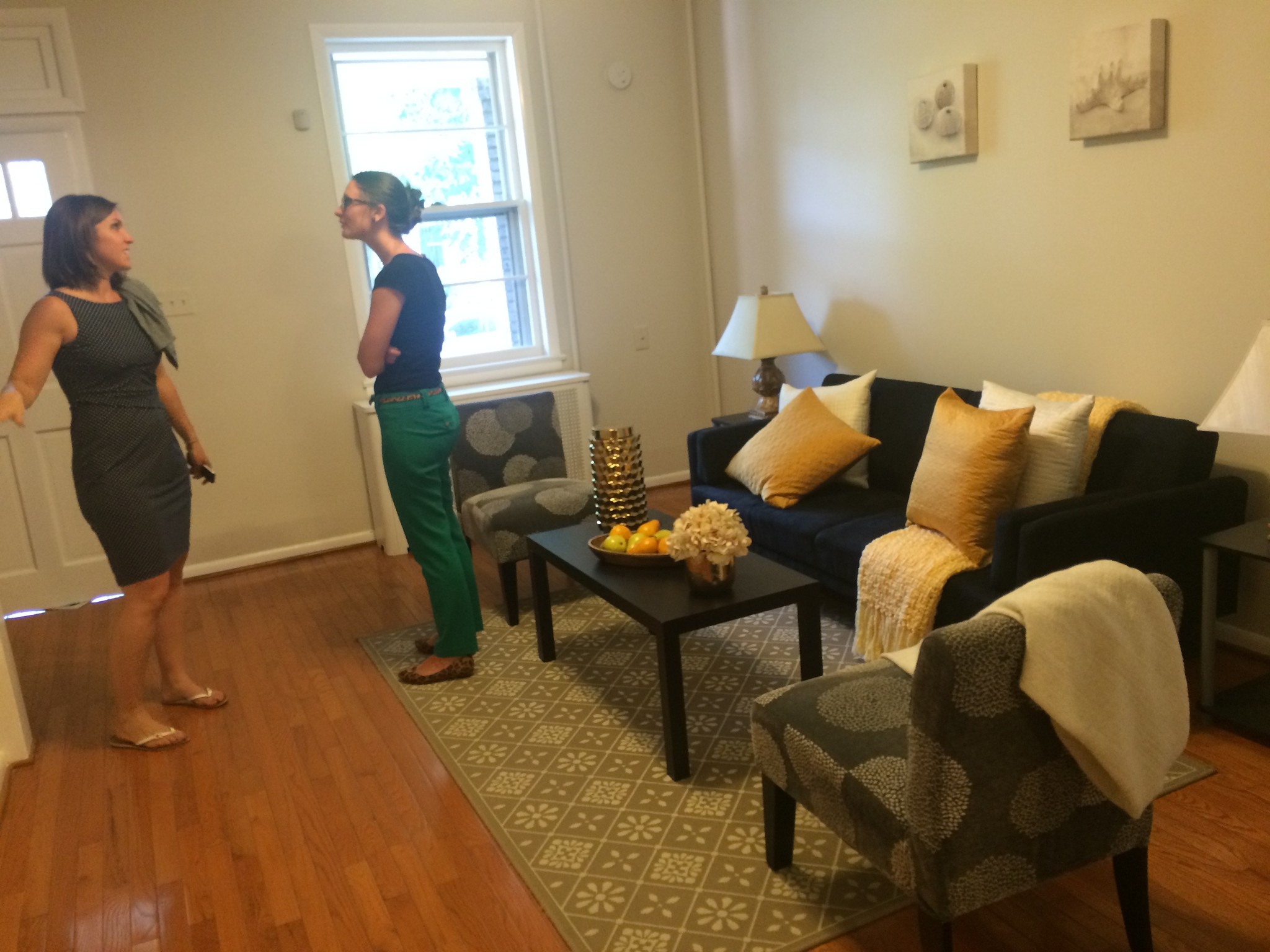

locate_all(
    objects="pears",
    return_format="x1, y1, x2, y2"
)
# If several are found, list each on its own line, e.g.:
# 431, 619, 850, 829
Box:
599, 519, 671, 554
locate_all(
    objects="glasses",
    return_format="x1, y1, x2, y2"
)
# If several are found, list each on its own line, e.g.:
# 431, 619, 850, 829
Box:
343, 193, 378, 209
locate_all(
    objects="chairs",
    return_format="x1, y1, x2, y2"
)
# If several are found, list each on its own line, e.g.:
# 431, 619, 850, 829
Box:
748, 560, 1184, 952
446, 389, 594, 626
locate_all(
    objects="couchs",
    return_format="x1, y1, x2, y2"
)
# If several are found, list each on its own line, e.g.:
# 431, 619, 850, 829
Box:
685, 373, 1249, 640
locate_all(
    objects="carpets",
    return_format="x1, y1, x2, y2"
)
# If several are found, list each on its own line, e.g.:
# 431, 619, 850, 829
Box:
353, 586, 1215, 952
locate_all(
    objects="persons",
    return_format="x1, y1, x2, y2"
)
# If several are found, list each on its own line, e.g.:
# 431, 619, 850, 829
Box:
334, 171, 485, 686
0, 195, 230, 751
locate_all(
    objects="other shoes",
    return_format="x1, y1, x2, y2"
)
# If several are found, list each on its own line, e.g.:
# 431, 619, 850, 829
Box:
398, 655, 475, 683
416, 636, 438, 654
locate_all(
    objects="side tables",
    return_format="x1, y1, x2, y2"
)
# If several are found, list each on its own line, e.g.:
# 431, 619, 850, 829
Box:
711, 412, 756, 428
1197, 518, 1270, 746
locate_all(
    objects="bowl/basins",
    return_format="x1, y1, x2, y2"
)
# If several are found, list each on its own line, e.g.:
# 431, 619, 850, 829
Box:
587, 531, 685, 567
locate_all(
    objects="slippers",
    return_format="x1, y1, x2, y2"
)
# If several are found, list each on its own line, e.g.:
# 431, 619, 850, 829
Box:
163, 687, 228, 709
108, 727, 189, 751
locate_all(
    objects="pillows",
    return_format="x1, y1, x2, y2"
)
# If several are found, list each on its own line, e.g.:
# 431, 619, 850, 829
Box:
725, 386, 884, 509
904, 387, 1036, 568
977, 379, 1096, 516
777, 369, 878, 492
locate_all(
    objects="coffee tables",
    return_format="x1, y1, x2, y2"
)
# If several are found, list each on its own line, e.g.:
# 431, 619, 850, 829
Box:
524, 508, 824, 783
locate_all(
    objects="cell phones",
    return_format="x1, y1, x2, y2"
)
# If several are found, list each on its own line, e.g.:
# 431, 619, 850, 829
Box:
186, 453, 215, 483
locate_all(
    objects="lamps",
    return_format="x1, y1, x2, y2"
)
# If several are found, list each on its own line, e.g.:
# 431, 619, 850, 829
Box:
710, 285, 827, 420
1197, 324, 1269, 440
